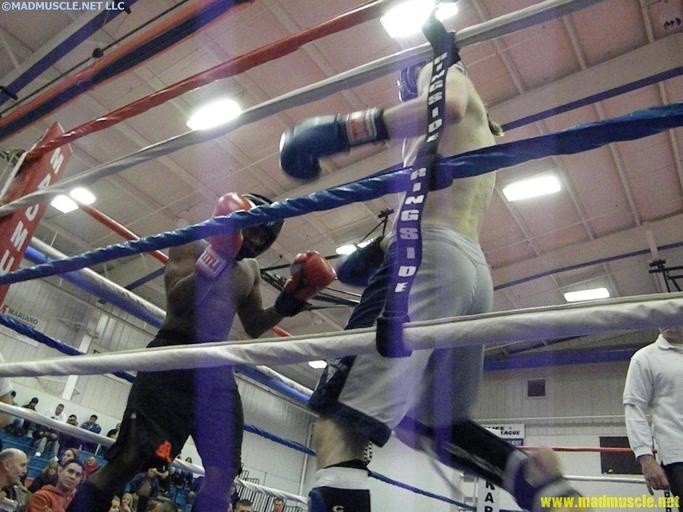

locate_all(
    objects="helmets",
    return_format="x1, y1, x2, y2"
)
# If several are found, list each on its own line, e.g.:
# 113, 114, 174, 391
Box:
240, 193, 283, 265
399, 60, 467, 102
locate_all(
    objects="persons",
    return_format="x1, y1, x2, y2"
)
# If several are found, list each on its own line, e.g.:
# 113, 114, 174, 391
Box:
65, 193, 336, 512
277, 54, 586, 512
1, 391, 287, 512
624, 328, 683, 512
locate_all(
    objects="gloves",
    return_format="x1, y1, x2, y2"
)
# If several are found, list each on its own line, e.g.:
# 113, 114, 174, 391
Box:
338, 234, 389, 286
194, 192, 254, 283
279, 106, 390, 182
275, 249, 337, 317
